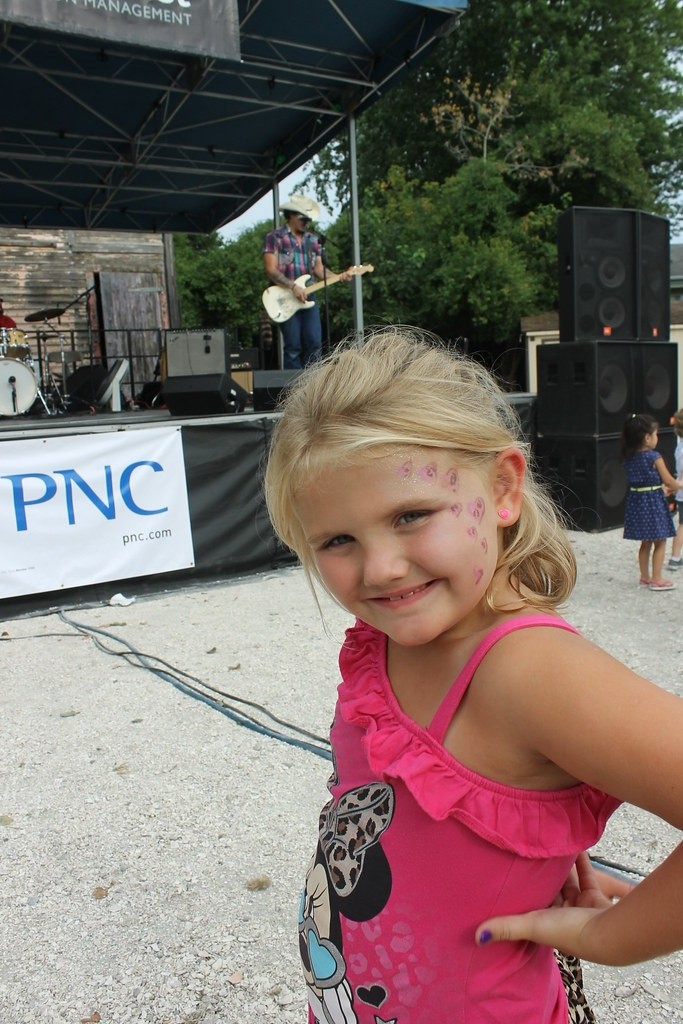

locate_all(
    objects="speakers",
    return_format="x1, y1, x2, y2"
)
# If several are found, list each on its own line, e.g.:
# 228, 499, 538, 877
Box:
46, 364, 127, 412
228, 348, 259, 395
165, 327, 231, 378
253, 369, 307, 411
500, 206, 683, 533
160, 374, 249, 416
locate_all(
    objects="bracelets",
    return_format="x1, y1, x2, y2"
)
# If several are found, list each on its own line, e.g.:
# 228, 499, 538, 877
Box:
291, 284, 296, 290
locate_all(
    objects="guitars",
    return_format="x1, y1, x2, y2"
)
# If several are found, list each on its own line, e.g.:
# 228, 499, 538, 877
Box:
261, 263, 374, 325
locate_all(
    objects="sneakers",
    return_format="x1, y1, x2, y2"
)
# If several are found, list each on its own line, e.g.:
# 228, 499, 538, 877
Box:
668, 557, 683, 570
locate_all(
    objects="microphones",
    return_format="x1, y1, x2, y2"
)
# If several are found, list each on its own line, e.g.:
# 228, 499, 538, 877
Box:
205, 344, 210, 353
8, 376, 16, 383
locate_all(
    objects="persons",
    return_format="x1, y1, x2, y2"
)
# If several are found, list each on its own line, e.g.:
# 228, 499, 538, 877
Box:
0, 298, 16, 328
262, 193, 352, 411
623, 408, 683, 591
264, 321, 683, 1024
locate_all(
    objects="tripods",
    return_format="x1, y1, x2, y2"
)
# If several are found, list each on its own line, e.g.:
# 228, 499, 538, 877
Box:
39, 317, 101, 416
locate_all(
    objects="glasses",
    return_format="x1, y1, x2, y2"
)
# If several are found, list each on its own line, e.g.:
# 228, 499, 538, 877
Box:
297, 215, 311, 223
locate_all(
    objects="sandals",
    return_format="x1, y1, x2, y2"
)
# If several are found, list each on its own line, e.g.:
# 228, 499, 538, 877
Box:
649, 580, 676, 590
638, 580, 650, 589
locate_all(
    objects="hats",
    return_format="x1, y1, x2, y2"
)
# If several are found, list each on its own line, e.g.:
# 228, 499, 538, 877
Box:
278, 195, 322, 223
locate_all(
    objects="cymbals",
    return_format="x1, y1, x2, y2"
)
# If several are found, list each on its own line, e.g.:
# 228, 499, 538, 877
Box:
24, 308, 66, 322
29, 335, 59, 339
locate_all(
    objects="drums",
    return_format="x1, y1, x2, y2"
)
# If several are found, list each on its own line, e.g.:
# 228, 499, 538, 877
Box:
0, 357, 39, 417
1, 327, 29, 358
